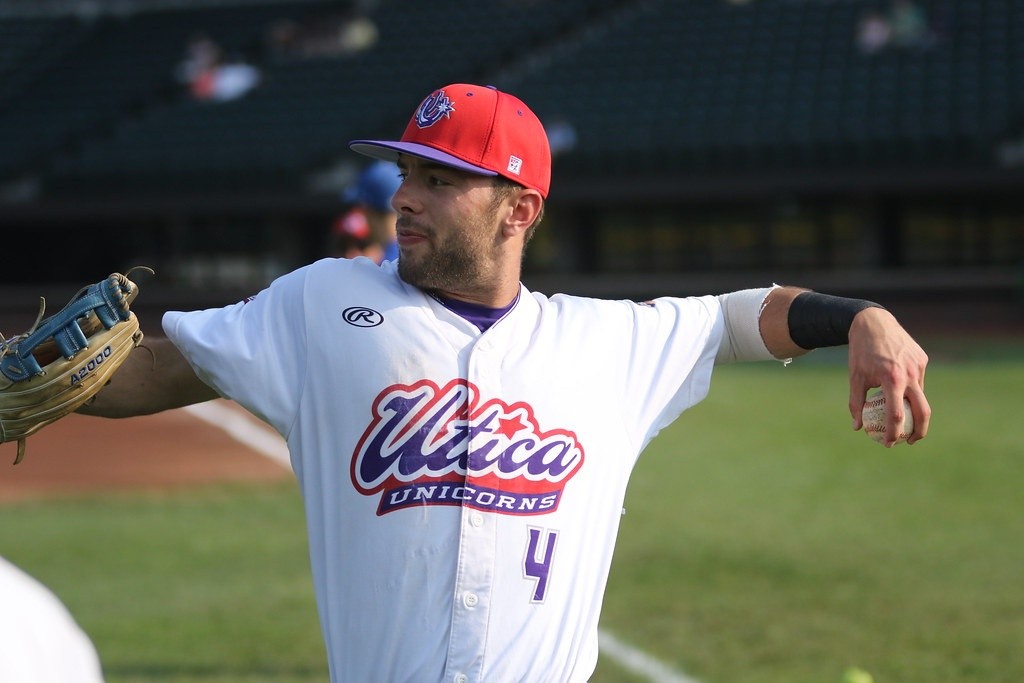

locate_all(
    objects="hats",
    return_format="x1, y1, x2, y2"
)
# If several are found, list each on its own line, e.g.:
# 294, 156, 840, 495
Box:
349, 83, 551, 200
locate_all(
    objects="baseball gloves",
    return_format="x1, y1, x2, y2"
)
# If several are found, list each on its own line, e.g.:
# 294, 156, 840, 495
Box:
0, 272, 146, 445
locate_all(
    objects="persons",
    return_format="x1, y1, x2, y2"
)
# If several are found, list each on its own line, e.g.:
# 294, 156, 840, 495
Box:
0, 83, 931, 683
340, 157, 402, 265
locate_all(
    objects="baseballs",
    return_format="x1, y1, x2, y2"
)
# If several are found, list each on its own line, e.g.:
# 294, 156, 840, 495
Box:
861, 389, 916, 447
